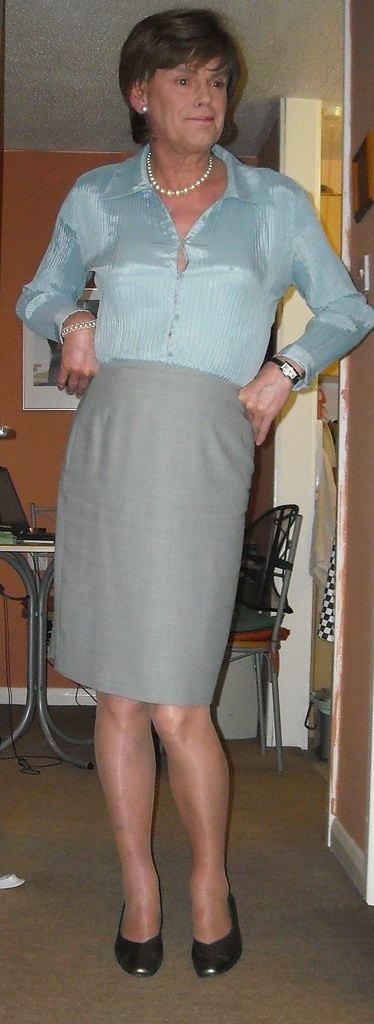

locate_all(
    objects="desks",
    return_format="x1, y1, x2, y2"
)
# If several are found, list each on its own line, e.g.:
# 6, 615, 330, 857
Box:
0, 532, 99, 773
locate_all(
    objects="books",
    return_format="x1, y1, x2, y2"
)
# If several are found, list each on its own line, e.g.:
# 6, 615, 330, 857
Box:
0, 534, 17, 545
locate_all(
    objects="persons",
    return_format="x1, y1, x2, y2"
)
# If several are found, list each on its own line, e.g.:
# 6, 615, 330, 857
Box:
13, 10, 374, 977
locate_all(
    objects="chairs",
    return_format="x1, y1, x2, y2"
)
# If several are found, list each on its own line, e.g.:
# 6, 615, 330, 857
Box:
155, 503, 302, 775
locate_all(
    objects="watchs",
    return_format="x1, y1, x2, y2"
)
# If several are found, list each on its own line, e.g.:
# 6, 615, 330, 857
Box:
270, 358, 300, 385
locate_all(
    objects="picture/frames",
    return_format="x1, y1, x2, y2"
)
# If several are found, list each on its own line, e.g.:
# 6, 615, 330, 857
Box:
20, 284, 100, 413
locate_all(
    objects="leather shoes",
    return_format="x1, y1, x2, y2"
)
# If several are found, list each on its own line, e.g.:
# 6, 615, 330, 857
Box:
190, 892, 243, 978
114, 900, 164, 976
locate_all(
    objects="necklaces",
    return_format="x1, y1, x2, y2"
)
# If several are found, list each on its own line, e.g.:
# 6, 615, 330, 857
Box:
147, 152, 213, 196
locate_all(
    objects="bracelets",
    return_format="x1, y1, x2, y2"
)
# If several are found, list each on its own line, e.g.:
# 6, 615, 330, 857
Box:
61, 319, 98, 338
